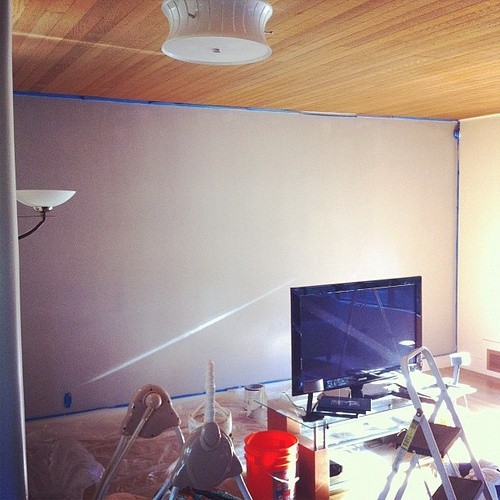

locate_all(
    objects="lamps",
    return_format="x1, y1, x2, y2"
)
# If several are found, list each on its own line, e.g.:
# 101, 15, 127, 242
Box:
16, 190, 76, 239
160, 0, 274, 66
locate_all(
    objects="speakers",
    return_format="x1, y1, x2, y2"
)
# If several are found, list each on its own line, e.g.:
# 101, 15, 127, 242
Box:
446, 352, 471, 387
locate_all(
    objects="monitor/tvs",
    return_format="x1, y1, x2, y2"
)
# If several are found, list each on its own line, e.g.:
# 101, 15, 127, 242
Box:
289, 275, 422, 402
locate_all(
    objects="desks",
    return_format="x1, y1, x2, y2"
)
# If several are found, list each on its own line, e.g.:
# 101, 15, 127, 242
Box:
253, 381, 477, 500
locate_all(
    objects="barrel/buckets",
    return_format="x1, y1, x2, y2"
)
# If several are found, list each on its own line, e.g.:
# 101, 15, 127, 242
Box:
244, 430, 301, 500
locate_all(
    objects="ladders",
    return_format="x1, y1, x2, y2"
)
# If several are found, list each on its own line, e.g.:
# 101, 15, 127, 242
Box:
378, 347, 493, 500
